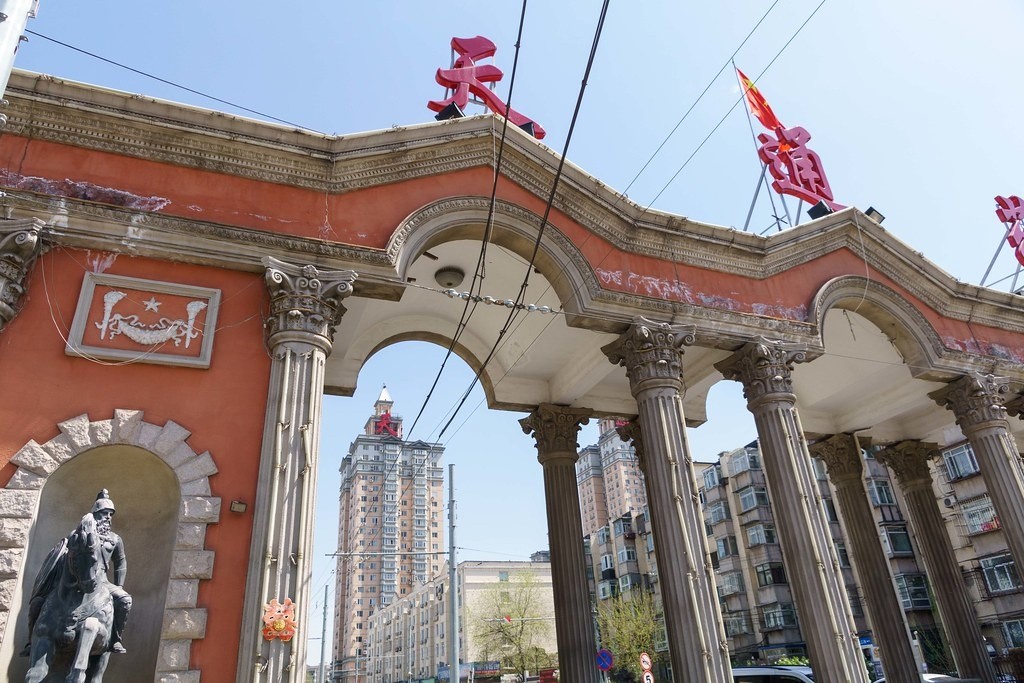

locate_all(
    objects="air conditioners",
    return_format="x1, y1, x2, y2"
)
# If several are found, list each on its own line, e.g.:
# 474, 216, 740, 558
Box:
942, 494, 956, 508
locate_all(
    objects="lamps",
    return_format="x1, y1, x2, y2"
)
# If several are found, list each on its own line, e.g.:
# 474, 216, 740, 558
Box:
231, 500, 248, 513
434, 101, 465, 121
520, 121, 536, 138
865, 207, 886, 225
805, 198, 833, 220
432, 267, 465, 289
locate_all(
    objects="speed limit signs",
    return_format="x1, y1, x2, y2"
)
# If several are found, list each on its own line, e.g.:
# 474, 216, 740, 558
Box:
642, 670, 655, 683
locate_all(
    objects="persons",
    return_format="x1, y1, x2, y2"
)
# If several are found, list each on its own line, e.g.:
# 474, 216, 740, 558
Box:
20, 488, 132, 658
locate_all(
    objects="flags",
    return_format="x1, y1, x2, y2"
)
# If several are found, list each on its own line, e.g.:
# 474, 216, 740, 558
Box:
737, 69, 790, 152
